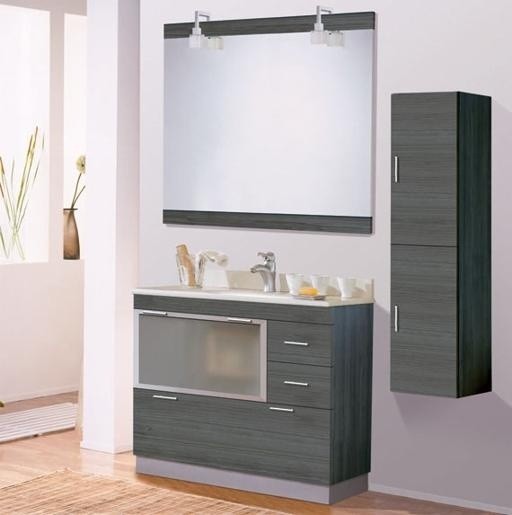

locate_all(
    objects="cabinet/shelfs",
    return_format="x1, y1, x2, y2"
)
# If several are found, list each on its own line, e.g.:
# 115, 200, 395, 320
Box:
135, 387, 372, 505
390, 91, 496, 400
134, 295, 373, 409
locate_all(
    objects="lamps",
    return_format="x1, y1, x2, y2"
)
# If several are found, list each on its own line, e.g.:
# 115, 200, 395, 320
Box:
187, 10, 211, 48
309, 7, 334, 46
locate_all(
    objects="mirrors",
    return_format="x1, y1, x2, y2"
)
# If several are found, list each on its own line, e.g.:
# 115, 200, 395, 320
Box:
161, 10, 376, 237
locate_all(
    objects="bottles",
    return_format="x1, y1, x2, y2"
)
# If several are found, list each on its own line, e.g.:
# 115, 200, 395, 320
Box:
174, 244, 196, 289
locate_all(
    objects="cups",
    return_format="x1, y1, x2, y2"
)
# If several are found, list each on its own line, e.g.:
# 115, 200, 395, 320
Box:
283, 273, 357, 299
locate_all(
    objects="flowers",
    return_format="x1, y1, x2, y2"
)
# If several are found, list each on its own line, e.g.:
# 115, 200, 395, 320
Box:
70, 154, 86, 209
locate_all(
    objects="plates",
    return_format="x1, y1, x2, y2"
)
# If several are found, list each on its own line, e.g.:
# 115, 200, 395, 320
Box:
290, 294, 325, 301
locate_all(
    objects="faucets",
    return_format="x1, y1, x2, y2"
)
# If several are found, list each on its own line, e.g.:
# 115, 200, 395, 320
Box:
248, 250, 276, 292
